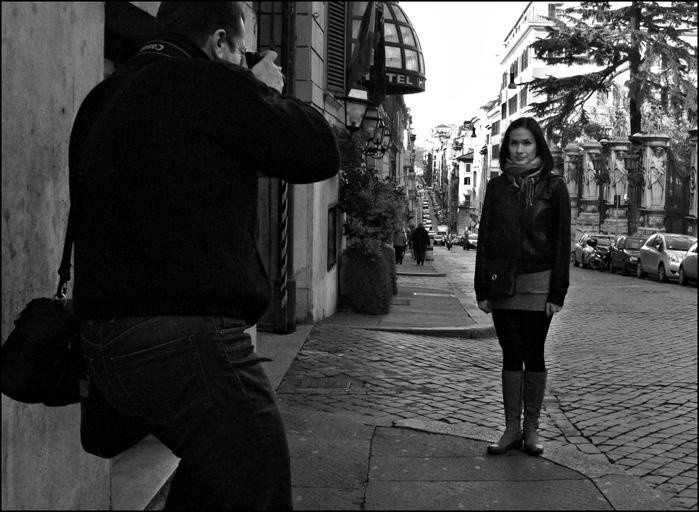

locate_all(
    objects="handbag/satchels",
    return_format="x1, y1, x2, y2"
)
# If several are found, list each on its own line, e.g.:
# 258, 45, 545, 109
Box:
485, 260, 516, 299
0, 299, 83, 407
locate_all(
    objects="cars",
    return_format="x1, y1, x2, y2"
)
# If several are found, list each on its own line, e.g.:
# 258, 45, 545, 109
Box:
417, 184, 478, 250
570, 232, 698, 286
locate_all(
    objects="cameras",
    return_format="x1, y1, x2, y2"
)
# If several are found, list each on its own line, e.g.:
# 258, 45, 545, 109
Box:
244, 51, 262, 69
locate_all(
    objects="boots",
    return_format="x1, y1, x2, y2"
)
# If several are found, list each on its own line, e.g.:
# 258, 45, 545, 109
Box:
486, 370, 523, 454
521, 371, 548, 454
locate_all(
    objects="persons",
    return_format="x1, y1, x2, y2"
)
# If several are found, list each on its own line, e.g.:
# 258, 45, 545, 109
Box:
462, 226, 470, 251
473, 116, 573, 457
68, 1, 343, 511
392, 220, 435, 267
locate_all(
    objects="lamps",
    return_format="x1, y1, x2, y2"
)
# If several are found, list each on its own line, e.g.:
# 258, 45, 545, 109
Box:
323, 87, 395, 160
